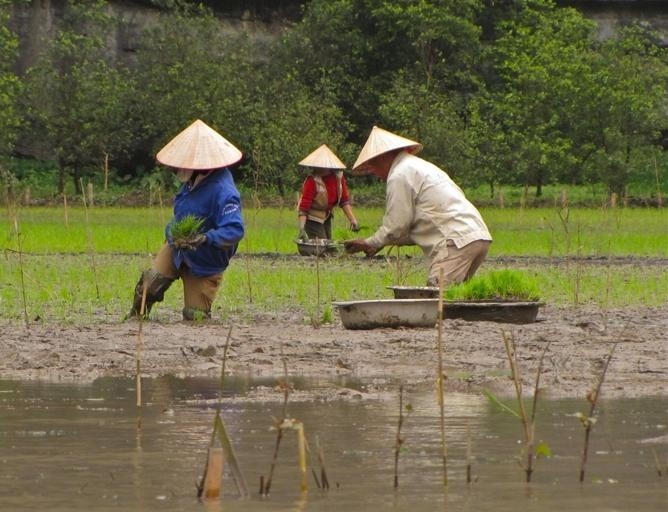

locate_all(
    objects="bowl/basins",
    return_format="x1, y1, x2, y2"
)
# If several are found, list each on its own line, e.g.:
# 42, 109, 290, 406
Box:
295, 237, 342, 256
331, 285, 547, 331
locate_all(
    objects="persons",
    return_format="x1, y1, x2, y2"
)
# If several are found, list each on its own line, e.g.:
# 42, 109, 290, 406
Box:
120, 117, 246, 324
295, 143, 361, 257
344, 125, 493, 285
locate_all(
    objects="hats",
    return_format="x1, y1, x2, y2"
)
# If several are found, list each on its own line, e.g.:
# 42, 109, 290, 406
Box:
298, 144, 348, 171
352, 124, 423, 176
154, 118, 244, 169
